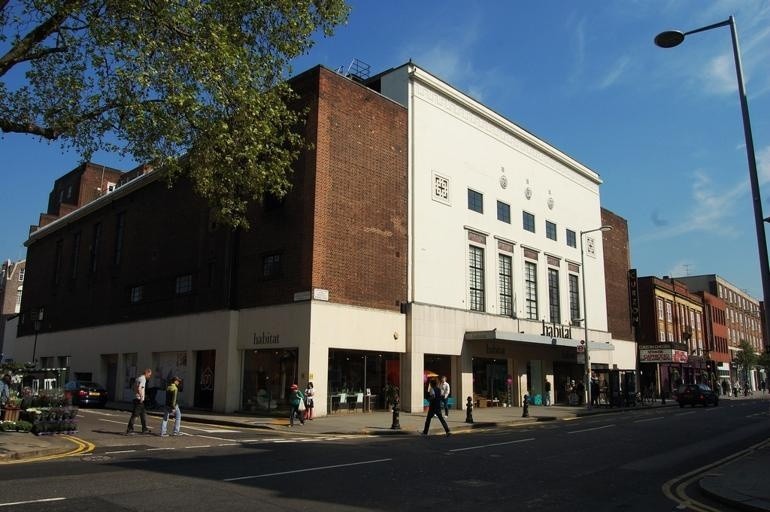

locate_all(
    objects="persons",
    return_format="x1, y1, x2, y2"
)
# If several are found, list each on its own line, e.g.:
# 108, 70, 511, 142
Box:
435, 376, 450, 418
709, 378, 749, 397
0, 374, 11, 409
160, 377, 183, 437
544, 379, 551, 406
420, 381, 452, 438
288, 384, 305, 427
126, 368, 152, 435
304, 382, 314, 420
570, 377, 600, 407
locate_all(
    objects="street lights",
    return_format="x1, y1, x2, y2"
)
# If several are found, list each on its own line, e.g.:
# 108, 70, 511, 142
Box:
30, 319, 41, 364
687, 346, 710, 385
653, 12, 769, 352
573, 225, 616, 410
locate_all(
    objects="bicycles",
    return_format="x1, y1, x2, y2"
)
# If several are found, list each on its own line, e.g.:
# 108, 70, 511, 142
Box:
606, 384, 657, 408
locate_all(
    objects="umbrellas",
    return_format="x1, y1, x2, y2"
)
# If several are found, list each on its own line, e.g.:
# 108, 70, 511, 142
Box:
425, 371, 438, 377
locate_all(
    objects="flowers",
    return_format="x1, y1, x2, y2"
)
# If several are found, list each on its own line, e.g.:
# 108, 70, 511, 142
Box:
1, 388, 80, 435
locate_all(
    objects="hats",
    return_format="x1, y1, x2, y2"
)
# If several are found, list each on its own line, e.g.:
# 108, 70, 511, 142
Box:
171, 377, 182, 381
291, 384, 298, 390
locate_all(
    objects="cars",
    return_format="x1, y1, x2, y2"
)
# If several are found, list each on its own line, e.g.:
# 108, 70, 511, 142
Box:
677, 384, 719, 409
65, 381, 110, 409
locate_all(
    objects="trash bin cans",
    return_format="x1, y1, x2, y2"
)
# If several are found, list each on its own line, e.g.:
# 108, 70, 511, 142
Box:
534, 393, 543, 405
